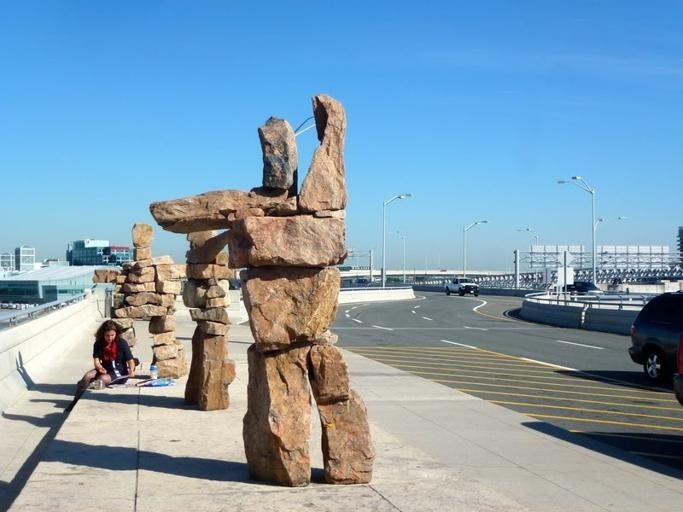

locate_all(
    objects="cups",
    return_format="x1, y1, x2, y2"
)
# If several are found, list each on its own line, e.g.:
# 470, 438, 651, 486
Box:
95, 379, 104, 390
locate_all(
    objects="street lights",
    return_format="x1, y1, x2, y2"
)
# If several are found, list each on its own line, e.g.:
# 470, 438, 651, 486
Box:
517, 227, 539, 245
382, 193, 412, 288
558, 176, 603, 285
464, 220, 488, 277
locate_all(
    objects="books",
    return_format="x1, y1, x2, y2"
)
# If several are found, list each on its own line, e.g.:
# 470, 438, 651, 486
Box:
136, 379, 156, 386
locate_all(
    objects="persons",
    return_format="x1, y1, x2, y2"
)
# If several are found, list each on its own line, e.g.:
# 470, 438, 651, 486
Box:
64, 321, 136, 412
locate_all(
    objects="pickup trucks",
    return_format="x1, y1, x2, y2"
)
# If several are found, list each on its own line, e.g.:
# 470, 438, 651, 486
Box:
562, 282, 600, 295
444, 277, 480, 296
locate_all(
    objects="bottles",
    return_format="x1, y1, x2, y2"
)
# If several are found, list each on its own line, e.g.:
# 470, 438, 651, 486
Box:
150, 366, 157, 380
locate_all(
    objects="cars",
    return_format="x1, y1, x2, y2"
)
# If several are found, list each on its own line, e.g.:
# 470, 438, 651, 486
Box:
629, 290, 683, 406
